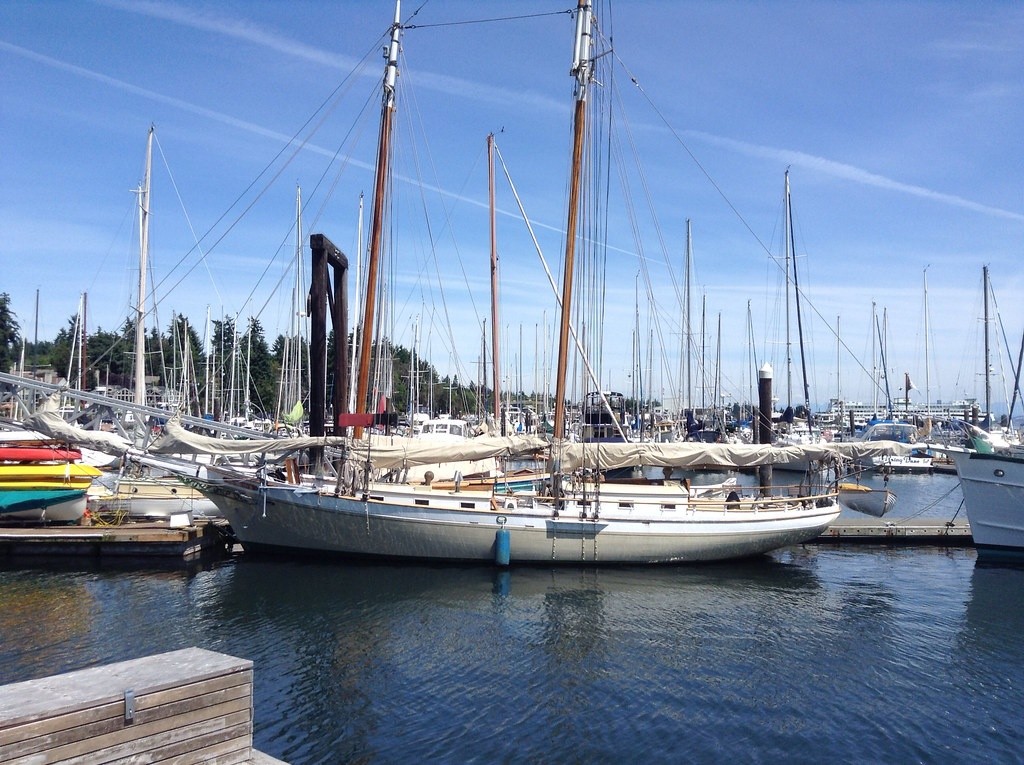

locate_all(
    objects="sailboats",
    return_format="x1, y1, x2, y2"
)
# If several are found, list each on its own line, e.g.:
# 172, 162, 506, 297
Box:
0, 3, 1024, 568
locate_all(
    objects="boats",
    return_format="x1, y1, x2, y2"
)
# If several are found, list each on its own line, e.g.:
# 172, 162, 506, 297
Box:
915, 415, 1024, 555
